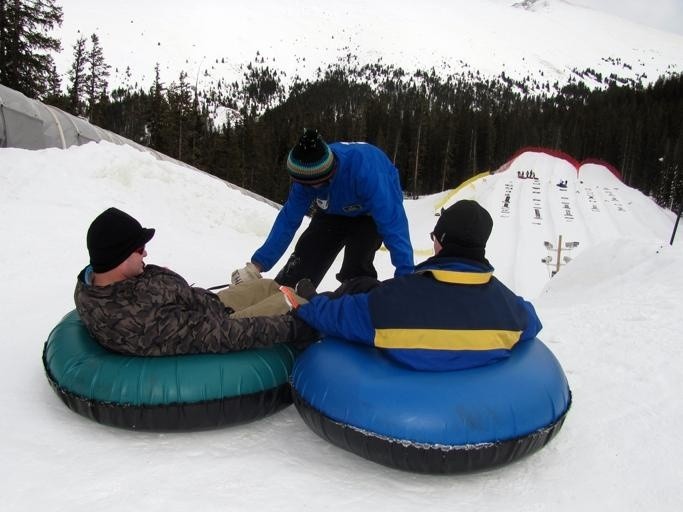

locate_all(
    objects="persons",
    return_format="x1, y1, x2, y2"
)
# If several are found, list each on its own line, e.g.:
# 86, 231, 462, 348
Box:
230, 128, 413, 290
294, 200, 542, 373
74, 207, 309, 357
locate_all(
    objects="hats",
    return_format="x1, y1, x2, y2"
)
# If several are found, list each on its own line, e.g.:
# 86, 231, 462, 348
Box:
87, 206, 156, 273
433, 199, 494, 249
286, 127, 336, 185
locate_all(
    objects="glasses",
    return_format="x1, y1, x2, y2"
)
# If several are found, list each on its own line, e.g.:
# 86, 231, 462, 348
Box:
133, 247, 145, 256
431, 233, 435, 239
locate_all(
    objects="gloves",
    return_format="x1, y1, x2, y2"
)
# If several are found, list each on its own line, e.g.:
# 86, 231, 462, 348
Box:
229, 262, 261, 289
280, 286, 298, 313
